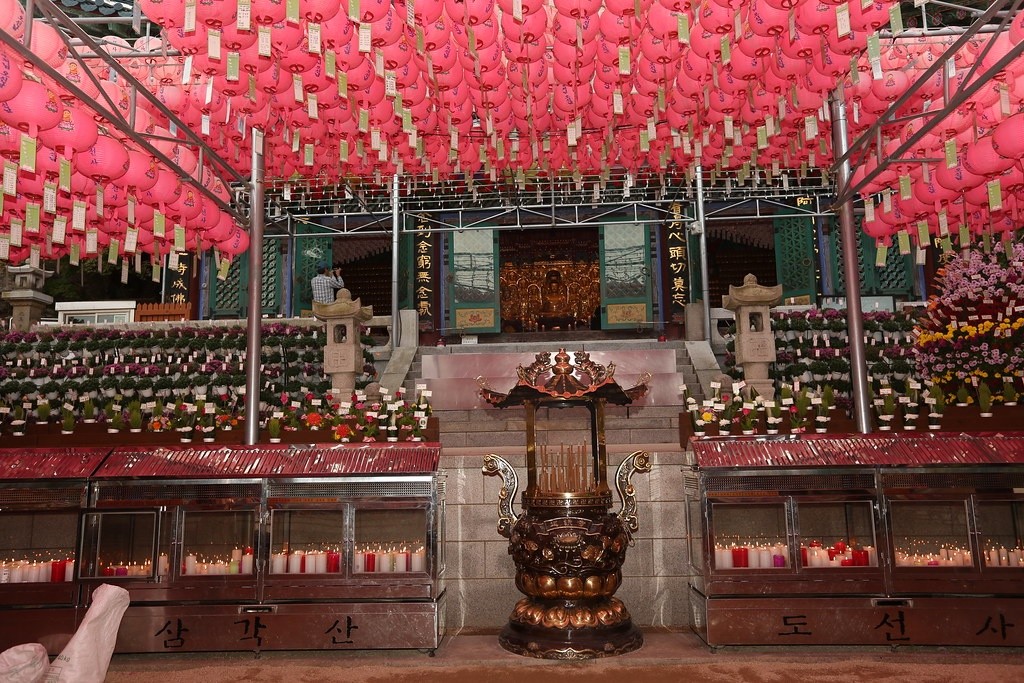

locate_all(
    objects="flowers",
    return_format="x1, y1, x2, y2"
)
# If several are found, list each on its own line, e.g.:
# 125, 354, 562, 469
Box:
723, 345, 914, 364
774, 307, 892, 321
0, 359, 325, 379
0, 320, 366, 341
147, 390, 434, 442
679, 384, 801, 430
913, 238, 1024, 406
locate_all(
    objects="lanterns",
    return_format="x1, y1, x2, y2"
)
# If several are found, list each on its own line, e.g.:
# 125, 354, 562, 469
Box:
1, 1, 1023, 274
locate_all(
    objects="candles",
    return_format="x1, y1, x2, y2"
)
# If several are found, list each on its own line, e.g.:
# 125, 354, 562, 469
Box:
801, 540, 877, 567
985, 546, 1024, 567
896, 545, 972, 567
271, 546, 343, 574
185, 545, 255, 575
102, 558, 152, 577
0, 558, 75, 583
715, 542, 789, 568
354, 541, 426, 574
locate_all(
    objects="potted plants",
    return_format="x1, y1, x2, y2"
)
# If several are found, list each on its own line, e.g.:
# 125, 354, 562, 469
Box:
0, 336, 376, 356
268, 418, 282, 443
724, 359, 911, 382
723, 320, 913, 340
796, 386, 809, 422
816, 382, 1018, 434
0, 376, 371, 436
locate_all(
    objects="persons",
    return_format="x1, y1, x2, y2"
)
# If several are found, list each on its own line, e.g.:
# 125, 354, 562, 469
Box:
498, 269, 600, 329
310, 263, 343, 314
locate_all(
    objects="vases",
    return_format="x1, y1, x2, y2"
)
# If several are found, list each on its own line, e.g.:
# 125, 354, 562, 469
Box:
742, 430, 754, 435
719, 431, 730, 436
203, 437, 216, 443
387, 437, 398, 442
694, 431, 705, 437
767, 429, 779, 435
180, 438, 193, 443
412, 437, 422, 442
